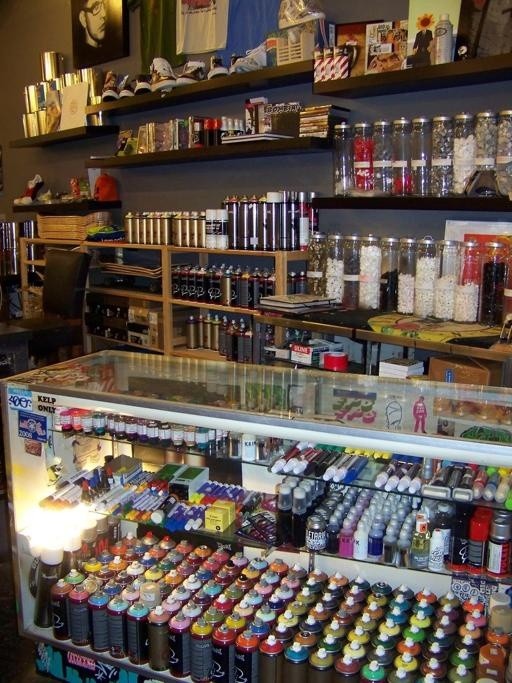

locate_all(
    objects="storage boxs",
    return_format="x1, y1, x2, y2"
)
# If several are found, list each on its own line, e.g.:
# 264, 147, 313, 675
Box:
425, 352, 504, 387
287, 337, 344, 366
124, 296, 201, 347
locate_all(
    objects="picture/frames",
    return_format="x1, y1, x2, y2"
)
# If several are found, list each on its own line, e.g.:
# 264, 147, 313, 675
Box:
332, 18, 385, 78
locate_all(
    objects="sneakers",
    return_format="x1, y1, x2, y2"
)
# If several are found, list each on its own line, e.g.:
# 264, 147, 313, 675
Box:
35, 210, 113, 241
277, 0, 327, 30
101, 70, 135, 102
230, 43, 268, 75
206, 54, 228, 79
12, 172, 45, 207
133, 57, 176, 94
176, 60, 205, 86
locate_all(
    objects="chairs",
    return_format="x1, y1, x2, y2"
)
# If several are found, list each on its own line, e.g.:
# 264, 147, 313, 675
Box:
6, 247, 94, 371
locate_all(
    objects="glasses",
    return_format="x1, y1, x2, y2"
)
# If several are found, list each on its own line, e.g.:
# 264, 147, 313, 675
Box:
81, 0, 106, 17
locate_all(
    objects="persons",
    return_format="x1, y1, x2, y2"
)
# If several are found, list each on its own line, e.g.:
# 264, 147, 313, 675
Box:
77, 0, 107, 66
411, 394, 427, 433
411, 16, 432, 66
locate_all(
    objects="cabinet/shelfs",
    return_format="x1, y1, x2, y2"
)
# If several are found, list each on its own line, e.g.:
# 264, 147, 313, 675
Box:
8, 121, 127, 214
310, 50, 511, 213
84, 56, 340, 172
2, 343, 511, 681
251, 310, 511, 394
17, 233, 315, 370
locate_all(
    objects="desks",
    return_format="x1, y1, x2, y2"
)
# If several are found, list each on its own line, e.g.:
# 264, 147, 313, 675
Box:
0, 313, 34, 379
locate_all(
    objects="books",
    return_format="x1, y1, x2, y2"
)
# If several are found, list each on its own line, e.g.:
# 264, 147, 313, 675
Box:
299, 104, 350, 138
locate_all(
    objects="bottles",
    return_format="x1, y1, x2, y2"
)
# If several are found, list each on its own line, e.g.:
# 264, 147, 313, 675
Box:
124, 111, 512, 369
31, 399, 511, 683
433, 11, 454, 64
